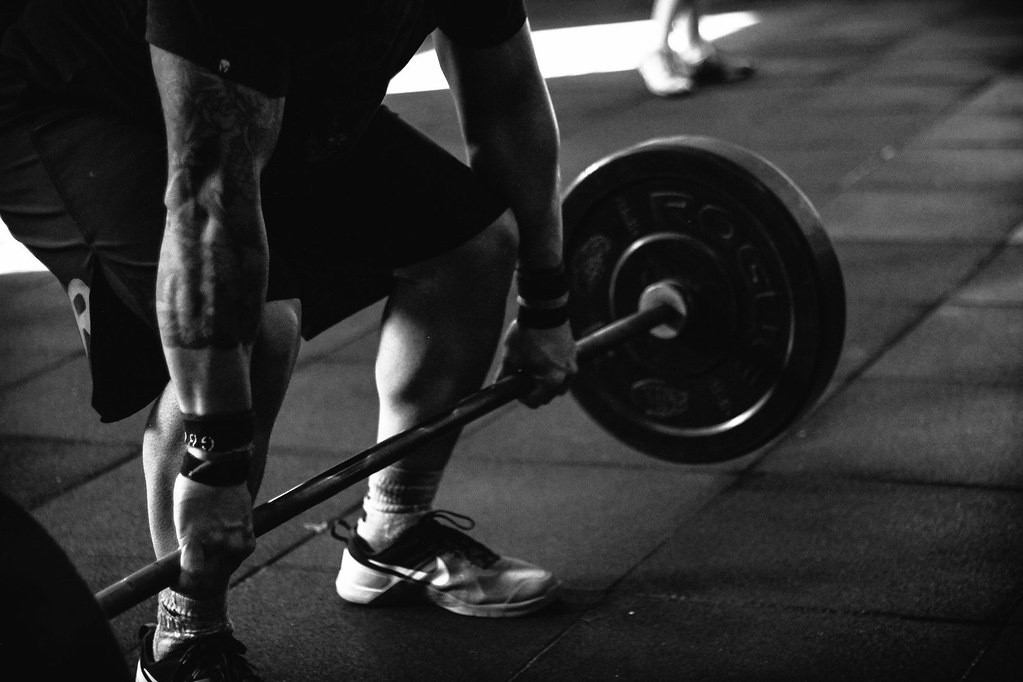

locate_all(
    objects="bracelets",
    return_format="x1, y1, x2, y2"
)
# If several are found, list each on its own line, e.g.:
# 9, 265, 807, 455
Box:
517, 305, 568, 330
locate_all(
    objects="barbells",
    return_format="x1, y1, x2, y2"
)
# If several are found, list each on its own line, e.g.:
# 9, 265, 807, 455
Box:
0, 134, 849, 682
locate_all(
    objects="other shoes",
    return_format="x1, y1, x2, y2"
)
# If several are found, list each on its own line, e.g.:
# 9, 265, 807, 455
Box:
636, 44, 694, 98
690, 41, 756, 84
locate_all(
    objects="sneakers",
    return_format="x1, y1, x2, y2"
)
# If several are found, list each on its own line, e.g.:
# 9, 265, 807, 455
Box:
329, 509, 563, 618
135, 623, 267, 682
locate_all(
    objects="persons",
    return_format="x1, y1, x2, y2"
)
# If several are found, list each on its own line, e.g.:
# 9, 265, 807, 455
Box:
635, 0, 754, 99
0, 0, 579, 682
